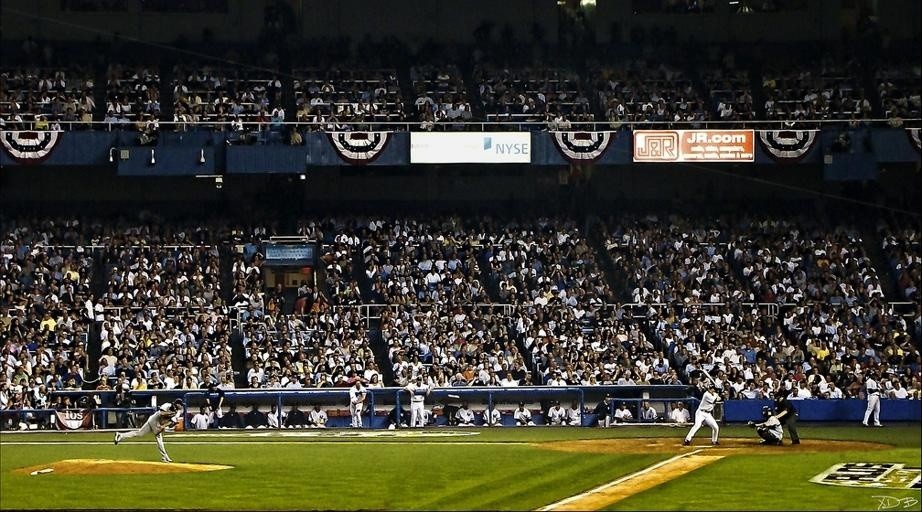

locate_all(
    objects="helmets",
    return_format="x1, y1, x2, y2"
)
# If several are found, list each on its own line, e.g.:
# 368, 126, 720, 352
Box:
761, 406, 774, 417
709, 384, 715, 388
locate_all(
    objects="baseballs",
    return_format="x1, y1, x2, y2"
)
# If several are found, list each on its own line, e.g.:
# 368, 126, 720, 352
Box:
449, 394, 460, 399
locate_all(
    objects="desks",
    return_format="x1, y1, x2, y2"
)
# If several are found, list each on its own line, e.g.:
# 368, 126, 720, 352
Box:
723, 398, 921, 422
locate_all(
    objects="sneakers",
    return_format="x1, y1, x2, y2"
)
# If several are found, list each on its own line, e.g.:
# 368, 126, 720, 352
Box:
684, 439, 689, 445
712, 442, 719, 444
114, 432, 120, 445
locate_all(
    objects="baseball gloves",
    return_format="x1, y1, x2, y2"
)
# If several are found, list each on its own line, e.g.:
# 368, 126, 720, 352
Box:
748, 421, 756, 428
167, 409, 176, 417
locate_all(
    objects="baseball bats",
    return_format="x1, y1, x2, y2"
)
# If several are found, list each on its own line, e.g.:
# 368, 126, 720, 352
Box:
702, 368, 724, 395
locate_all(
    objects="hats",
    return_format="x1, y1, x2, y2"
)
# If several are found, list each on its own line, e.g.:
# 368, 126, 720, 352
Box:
175, 399, 185, 406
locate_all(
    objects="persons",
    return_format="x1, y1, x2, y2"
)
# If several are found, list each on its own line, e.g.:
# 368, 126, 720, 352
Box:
114, 398, 183, 463
773, 393, 799, 444
1, 64, 922, 428
747, 404, 783, 445
683, 383, 719, 445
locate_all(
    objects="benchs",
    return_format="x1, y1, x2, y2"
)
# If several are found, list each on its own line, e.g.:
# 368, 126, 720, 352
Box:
0, 36, 922, 131
187, 413, 598, 429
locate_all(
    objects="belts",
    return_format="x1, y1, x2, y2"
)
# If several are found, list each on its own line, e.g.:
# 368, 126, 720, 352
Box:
701, 409, 710, 413
